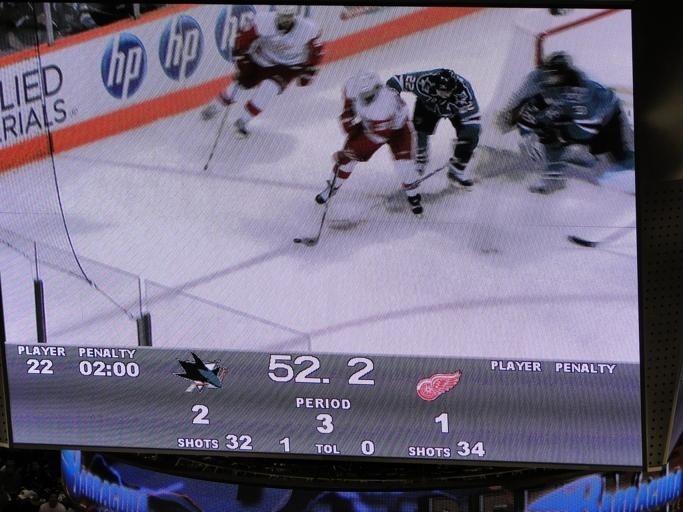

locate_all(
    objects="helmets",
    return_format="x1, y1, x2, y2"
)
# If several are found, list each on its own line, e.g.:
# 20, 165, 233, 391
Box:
540, 50, 575, 88
433, 68, 458, 98
278, 5, 297, 28
355, 66, 384, 106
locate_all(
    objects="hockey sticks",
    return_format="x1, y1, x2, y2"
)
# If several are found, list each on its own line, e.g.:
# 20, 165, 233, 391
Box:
203, 81, 237, 170
568, 222, 637, 247
327, 160, 455, 222
302, 160, 342, 247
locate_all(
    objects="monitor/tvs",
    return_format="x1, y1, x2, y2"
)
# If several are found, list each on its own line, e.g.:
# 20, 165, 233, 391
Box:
0, 0, 683, 491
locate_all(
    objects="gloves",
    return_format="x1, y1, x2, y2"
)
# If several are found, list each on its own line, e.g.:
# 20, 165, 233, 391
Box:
339, 111, 356, 132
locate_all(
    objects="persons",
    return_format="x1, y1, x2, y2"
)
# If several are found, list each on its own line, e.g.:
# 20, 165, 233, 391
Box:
498, 49, 635, 196
385, 67, 483, 186
0, 0, 47, 53
0, 447, 81, 510
197, 5, 325, 140
49, 0, 100, 42
314, 69, 423, 215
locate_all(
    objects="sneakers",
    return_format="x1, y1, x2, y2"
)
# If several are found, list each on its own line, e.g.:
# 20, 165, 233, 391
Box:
407, 191, 424, 215
314, 180, 337, 204
529, 170, 567, 194
447, 168, 474, 188
198, 102, 251, 137
